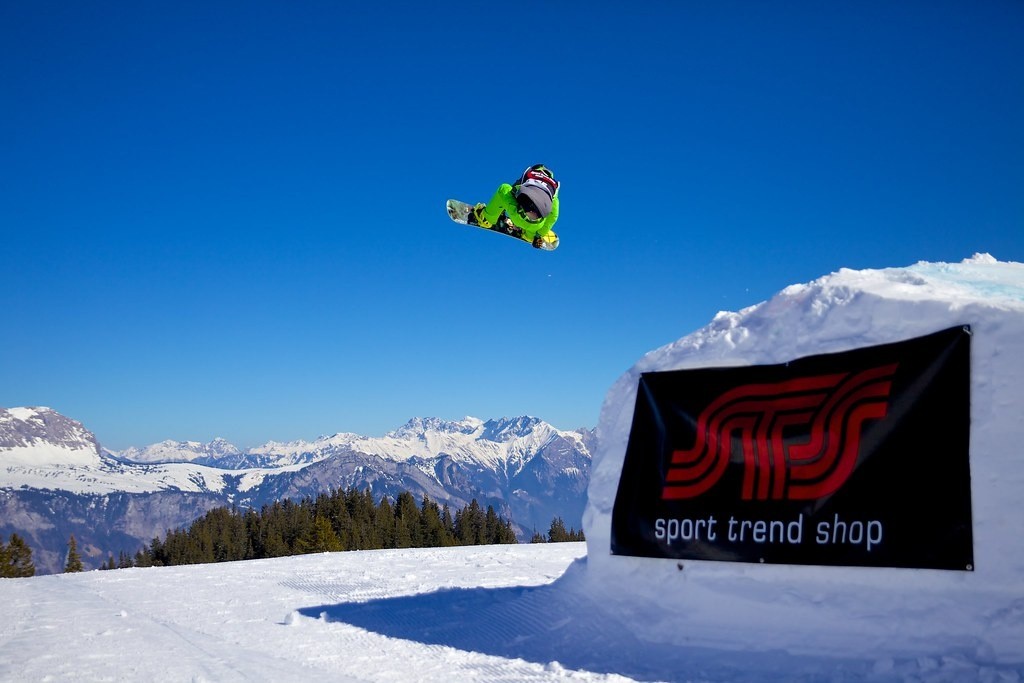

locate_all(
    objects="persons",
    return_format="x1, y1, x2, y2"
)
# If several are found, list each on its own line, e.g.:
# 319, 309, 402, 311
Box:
474, 164, 560, 243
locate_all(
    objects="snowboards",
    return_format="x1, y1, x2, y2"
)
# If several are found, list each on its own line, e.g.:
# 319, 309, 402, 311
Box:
444, 197, 560, 252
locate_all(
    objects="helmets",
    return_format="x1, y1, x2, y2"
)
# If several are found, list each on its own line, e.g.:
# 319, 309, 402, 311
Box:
530, 163, 554, 179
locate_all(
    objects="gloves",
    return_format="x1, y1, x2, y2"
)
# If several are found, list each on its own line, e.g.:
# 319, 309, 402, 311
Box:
532, 237, 543, 248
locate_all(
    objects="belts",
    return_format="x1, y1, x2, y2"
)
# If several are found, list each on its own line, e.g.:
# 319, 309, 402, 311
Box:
516, 202, 544, 225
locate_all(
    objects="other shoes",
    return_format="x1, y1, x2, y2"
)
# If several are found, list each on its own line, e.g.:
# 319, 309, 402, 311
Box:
474, 202, 493, 229
